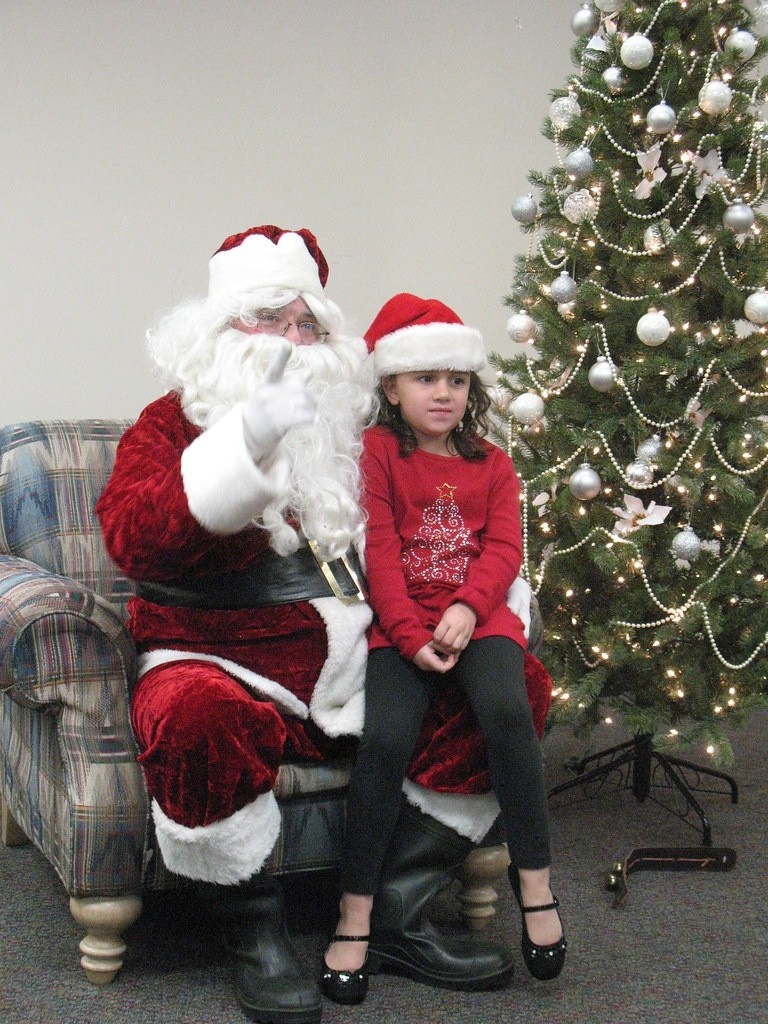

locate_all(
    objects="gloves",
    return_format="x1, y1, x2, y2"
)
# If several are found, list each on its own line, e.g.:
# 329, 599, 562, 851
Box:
504, 575, 531, 639
241, 346, 313, 464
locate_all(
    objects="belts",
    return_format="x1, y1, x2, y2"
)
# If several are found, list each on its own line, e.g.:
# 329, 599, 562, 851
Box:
134, 540, 372, 611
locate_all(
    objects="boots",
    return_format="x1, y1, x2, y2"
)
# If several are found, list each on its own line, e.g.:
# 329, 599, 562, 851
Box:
192, 862, 323, 1024
366, 789, 513, 990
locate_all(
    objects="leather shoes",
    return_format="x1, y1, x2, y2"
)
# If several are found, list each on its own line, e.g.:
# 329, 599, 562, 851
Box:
323, 933, 370, 1005
508, 862, 567, 980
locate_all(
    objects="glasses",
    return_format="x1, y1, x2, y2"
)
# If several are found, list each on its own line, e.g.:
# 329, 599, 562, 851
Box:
236, 314, 329, 345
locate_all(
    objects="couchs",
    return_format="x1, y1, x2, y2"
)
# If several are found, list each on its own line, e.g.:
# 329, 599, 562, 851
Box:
0, 420, 511, 987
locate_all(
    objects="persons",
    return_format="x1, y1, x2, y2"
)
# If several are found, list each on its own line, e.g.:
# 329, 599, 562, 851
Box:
94, 225, 553, 1022
317, 295, 567, 1005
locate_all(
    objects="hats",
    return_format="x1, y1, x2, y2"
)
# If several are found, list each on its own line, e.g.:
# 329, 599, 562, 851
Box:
209, 225, 348, 342
361, 293, 487, 381
